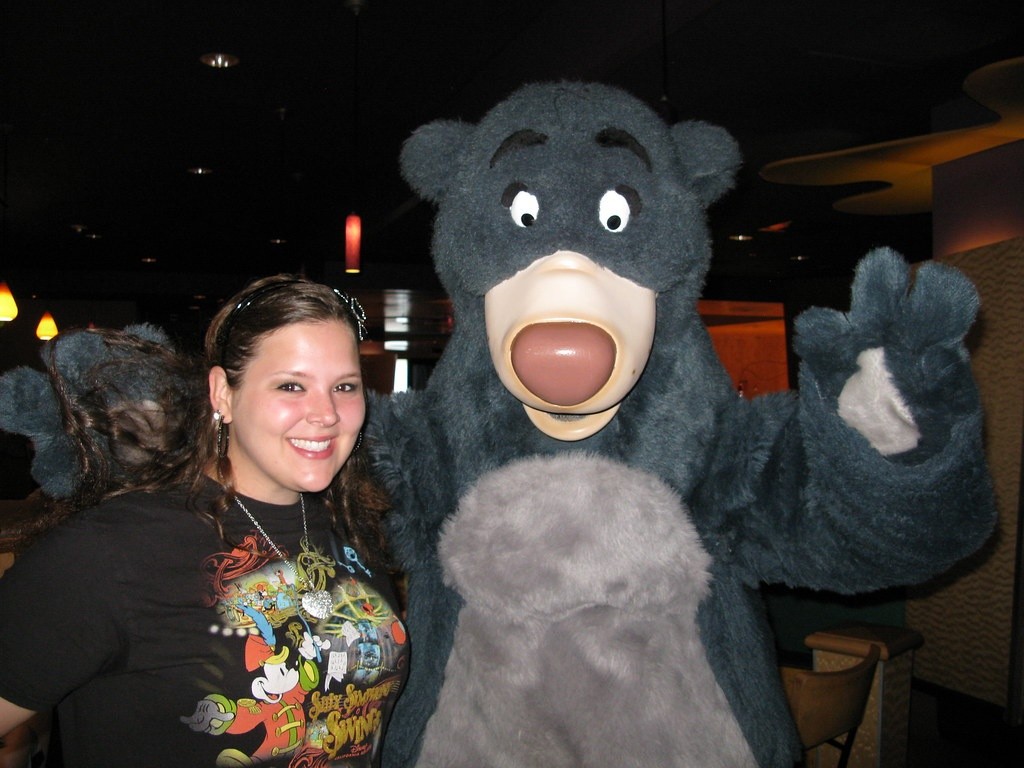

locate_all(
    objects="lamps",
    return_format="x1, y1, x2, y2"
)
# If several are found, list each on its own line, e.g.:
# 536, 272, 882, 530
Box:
36, 310, 58, 341
0, 279, 18, 322
345, 210, 362, 274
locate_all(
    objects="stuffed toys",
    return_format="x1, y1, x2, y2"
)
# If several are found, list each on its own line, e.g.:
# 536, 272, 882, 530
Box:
0, 80, 999, 768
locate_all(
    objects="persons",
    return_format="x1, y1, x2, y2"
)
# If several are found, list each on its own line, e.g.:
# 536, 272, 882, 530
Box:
0, 275, 411, 768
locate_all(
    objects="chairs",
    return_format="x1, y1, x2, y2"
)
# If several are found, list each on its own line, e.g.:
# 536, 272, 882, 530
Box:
779, 644, 880, 768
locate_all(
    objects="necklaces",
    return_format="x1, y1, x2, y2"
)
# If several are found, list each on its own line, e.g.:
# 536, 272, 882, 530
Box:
233, 492, 334, 620
